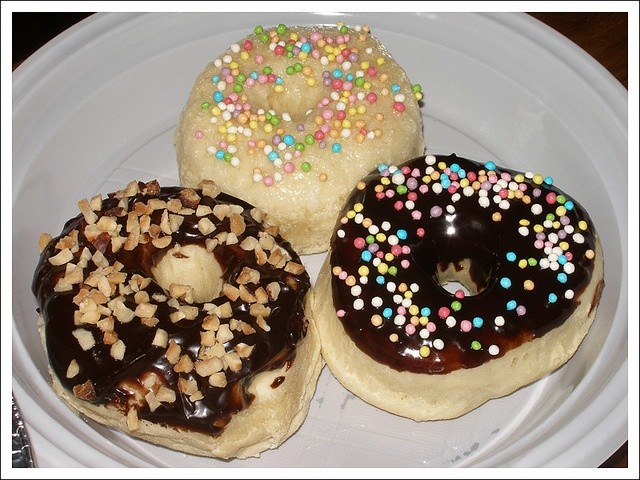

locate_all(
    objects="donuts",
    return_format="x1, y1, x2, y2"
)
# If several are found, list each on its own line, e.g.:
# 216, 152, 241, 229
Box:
32, 180, 326, 462
177, 23, 426, 256
313, 154, 604, 422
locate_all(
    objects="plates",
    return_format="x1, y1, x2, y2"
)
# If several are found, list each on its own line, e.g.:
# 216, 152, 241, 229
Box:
12, 12, 627, 468
